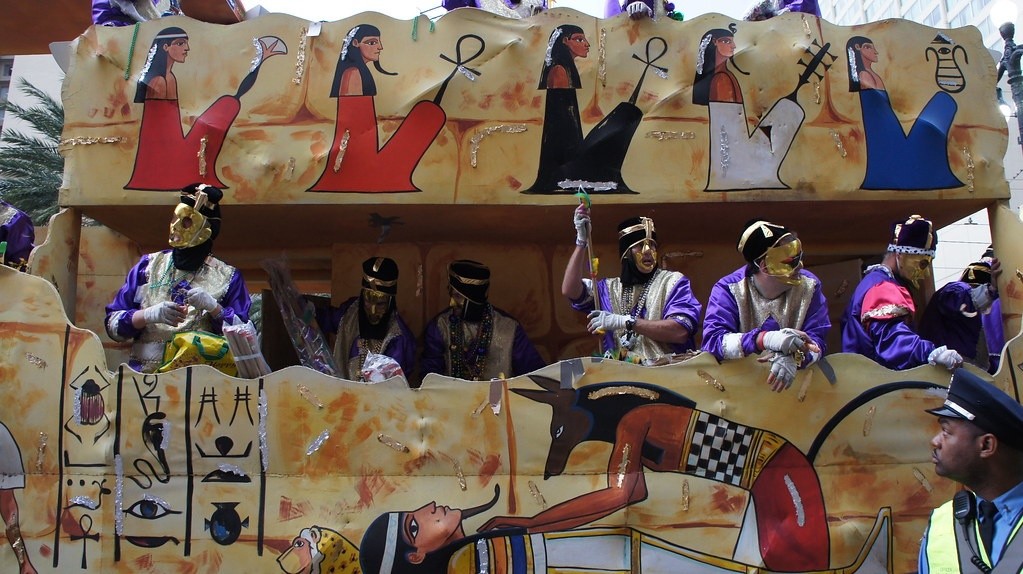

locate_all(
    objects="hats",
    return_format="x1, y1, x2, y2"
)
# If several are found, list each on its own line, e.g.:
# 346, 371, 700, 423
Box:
887, 215, 938, 259
180, 183, 223, 221
447, 259, 492, 305
736, 219, 792, 265
924, 368, 1023, 451
959, 261, 992, 285
360, 256, 399, 295
617, 217, 658, 263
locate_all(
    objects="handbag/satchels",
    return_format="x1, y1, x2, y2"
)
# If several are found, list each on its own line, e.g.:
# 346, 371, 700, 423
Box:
153, 331, 238, 377
361, 350, 409, 387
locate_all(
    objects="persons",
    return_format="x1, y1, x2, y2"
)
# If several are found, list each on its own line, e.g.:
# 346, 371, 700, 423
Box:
0, 200, 36, 272
441, 0, 557, 18
299, 256, 415, 387
104, 182, 251, 375
421, 259, 547, 381
840, 214, 964, 372
561, 204, 702, 366
92, 0, 183, 28
917, 369, 1023, 574
743, 0, 822, 21
701, 221, 832, 393
916, 243, 1005, 374
604, 0, 684, 21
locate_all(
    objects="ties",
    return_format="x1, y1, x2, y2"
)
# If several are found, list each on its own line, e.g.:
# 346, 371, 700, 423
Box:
979, 501, 999, 563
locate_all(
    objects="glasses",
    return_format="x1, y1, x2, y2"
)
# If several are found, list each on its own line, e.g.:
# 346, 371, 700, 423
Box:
782, 250, 804, 268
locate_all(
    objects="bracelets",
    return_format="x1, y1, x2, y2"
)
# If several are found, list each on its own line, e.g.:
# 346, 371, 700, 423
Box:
626, 316, 637, 331
216, 309, 224, 318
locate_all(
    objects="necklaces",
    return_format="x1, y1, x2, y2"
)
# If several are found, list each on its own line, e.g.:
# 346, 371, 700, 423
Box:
620, 268, 661, 351
150, 254, 204, 302
356, 339, 382, 382
449, 303, 494, 381
